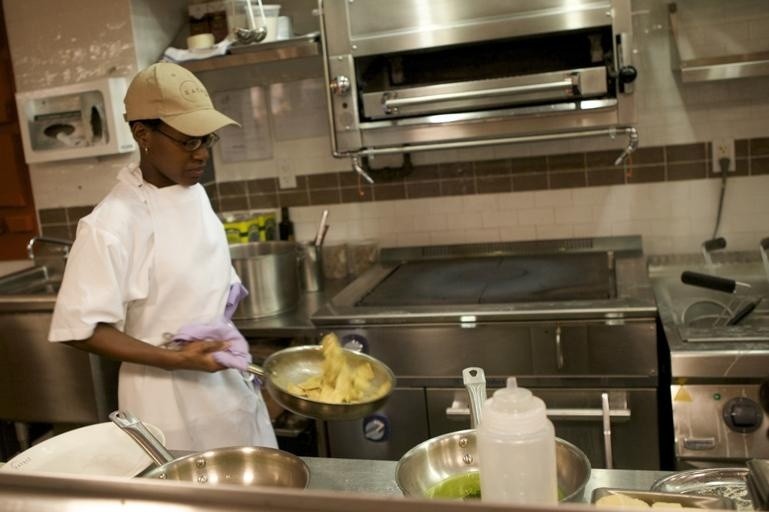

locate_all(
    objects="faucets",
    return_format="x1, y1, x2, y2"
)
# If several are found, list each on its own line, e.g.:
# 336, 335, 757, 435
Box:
24, 237, 76, 258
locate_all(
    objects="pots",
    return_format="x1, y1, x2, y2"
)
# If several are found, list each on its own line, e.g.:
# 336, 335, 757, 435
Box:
226, 240, 301, 321
395, 366, 590, 502
162, 333, 398, 420
110, 407, 313, 489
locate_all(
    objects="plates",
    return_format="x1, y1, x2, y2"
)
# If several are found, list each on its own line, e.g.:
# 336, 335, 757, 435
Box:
2, 420, 164, 479
653, 465, 753, 511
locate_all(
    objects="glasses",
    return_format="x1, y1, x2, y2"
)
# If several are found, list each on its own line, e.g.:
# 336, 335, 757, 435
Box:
155, 127, 221, 152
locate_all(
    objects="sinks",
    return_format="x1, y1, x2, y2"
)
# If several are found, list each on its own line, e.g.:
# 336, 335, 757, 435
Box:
0, 265, 66, 295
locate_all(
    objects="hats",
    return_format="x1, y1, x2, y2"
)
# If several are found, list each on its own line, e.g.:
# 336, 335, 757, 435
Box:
123, 62, 242, 137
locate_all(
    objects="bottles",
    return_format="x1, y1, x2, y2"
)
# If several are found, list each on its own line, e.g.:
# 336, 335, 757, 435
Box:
278, 205, 296, 240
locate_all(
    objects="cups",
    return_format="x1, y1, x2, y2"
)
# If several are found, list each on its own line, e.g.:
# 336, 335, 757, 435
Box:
186, 33, 215, 51
301, 244, 324, 294
244, 4, 281, 44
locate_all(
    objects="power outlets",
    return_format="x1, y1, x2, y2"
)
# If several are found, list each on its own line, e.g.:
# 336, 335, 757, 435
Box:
712, 136, 736, 173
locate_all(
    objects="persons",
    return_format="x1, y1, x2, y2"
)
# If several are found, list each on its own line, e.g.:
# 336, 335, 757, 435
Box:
46, 62, 281, 452
